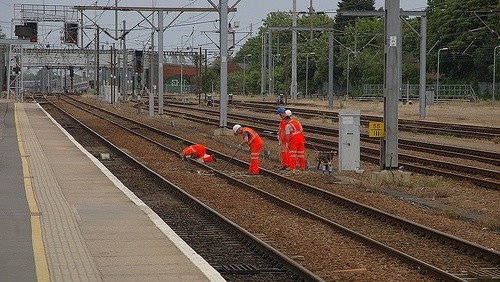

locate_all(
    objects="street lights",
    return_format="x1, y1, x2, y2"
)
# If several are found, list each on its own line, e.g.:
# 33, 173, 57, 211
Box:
437, 48, 448, 104
243, 54, 251, 96
272, 54, 281, 95
181, 63, 184, 94
492, 46, 500, 101
305, 52, 315, 99
346, 51, 358, 101
212, 56, 218, 95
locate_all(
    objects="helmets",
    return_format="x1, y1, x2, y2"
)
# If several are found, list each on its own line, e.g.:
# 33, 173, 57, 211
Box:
233, 125, 241, 134
277, 108, 285, 114
285, 110, 292, 115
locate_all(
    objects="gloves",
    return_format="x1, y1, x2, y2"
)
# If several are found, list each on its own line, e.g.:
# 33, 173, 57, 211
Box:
238, 144, 243, 149
279, 139, 282, 145
286, 143, 288, 148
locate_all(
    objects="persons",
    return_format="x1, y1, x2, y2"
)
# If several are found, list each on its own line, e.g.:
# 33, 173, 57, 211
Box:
179, 144, 218, 162
233, 124, 262, 175
277, 107, 305, 171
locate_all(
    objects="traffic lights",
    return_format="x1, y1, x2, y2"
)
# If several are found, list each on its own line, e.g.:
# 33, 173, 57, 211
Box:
30, 22, 37, 42
70, 23, 78, 43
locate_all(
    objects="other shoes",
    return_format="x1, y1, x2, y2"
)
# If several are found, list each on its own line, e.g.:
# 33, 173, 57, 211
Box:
212, 154, 216, 161
278, 166, 283, 170
286, 168, 290, 171
246, 173, 248, 175
